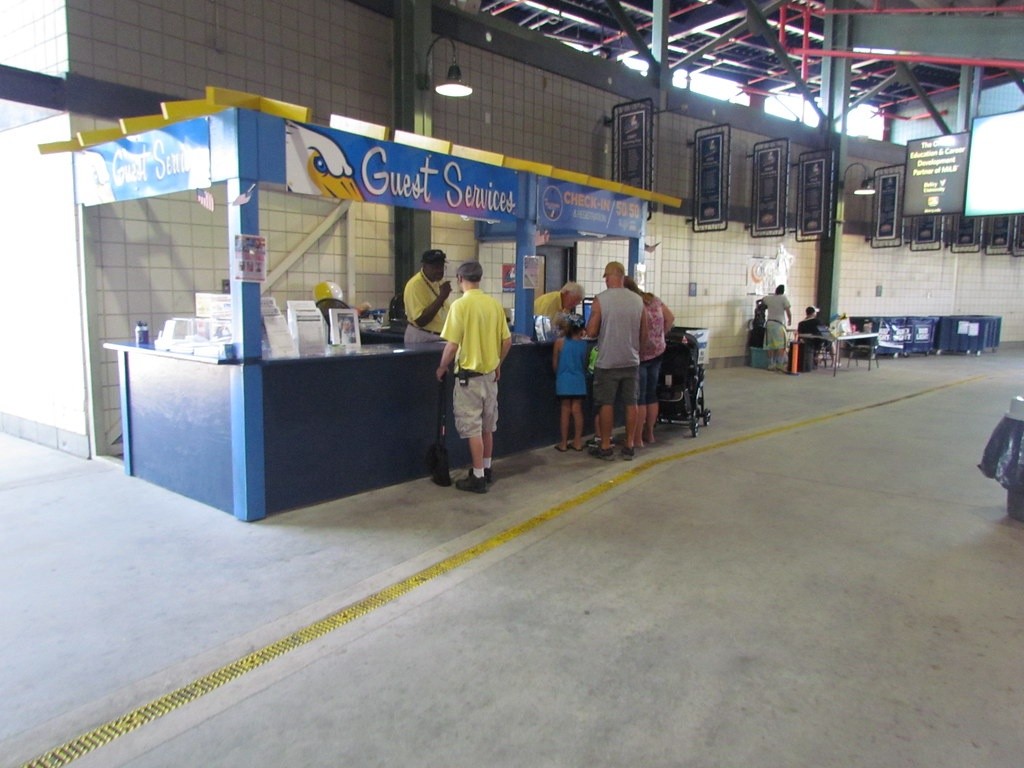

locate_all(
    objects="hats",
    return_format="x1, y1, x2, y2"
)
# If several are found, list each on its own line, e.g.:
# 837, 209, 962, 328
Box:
423, 249, 447, 264
806, 306, 820, 315
603, 262, 624, 277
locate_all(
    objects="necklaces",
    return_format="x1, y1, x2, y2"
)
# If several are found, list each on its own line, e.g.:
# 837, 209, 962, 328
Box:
421, 273, 445, 310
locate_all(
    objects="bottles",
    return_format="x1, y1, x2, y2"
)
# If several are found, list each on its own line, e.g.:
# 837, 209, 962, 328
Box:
134, 326, 149, 345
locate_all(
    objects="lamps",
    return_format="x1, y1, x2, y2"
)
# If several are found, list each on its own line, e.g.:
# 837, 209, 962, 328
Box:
840, 162, 877, 195
417, 35, 473, 98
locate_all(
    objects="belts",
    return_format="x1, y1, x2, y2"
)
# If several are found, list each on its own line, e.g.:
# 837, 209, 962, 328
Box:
455, 371, 484, 377
408, 321, 441, 335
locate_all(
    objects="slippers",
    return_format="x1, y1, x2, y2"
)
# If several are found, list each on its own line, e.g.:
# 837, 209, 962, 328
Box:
570, 442, 583, 451
555, 444, 567, 452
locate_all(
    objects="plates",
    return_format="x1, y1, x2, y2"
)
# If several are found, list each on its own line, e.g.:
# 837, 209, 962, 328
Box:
313, 281, 342, 303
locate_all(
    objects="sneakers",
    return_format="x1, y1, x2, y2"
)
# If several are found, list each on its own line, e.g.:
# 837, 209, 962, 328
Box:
585, 435, 601, 448
589, 447, 614, 460
470, 467, 493, 483
456, 473, 488, 494
609, 438, 616, 447
621, 447, 634, 459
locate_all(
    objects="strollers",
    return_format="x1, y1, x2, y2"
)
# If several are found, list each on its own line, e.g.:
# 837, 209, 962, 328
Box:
653, 331, 712, 438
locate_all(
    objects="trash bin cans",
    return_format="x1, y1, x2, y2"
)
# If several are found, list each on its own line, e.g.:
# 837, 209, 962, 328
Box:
1000, 394, 1024, 523
903, 316, 938, 356
848, 317, 907, 359
934, 314, 1001, 356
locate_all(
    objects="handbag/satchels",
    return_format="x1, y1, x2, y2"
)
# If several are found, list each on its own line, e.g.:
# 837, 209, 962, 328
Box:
433, 443, 451, 486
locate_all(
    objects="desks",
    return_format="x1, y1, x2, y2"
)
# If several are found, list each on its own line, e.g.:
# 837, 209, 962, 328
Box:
797, 333, 879, 377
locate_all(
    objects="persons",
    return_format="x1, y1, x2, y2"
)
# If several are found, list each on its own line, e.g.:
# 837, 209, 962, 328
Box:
534, 282, 585, 323
587, 261, 648, 460
436, 261, 512, 494
762, 284, 792, 372
552, 314, 589, 453
798, 306, 841, 368
624, 279, 675, 448
403, 249, 453, 344
585, 344, 615, 448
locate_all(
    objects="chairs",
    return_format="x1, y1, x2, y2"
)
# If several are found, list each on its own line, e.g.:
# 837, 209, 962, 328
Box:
799, 335, 837, 369
846, 334, 878, 371
